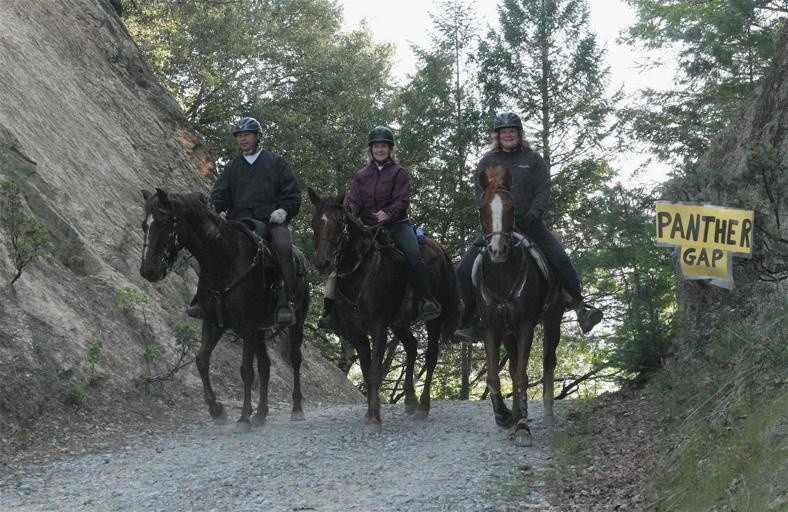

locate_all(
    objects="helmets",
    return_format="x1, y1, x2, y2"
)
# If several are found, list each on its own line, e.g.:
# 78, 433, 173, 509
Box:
232, 116, 263, 137
492, 112, 524, 132
366, 125, 395, 145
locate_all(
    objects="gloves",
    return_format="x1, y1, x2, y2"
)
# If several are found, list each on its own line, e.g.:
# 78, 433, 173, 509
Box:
267, 209, 288, 225
218, 208, 230, 221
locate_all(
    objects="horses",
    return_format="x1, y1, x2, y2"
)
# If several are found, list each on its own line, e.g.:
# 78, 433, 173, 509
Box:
138, 187, 310, 435
469, 165, 566, 447
306, 185, 461, 435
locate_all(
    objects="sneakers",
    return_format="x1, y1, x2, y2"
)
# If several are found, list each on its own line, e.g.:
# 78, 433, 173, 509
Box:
577, 307, 604, 334
276, 307, 293, 323
454, 326, 477, 341
184, 302, 202, 316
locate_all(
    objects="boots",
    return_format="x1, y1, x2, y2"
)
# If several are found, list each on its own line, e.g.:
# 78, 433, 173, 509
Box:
411, 263, 437, 313
318, 306, 334, 329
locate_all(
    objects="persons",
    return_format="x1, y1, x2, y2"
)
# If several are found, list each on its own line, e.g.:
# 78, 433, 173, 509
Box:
184, 117, 302, 324
451, 113, 602, 344
317, 126, 442, 332
